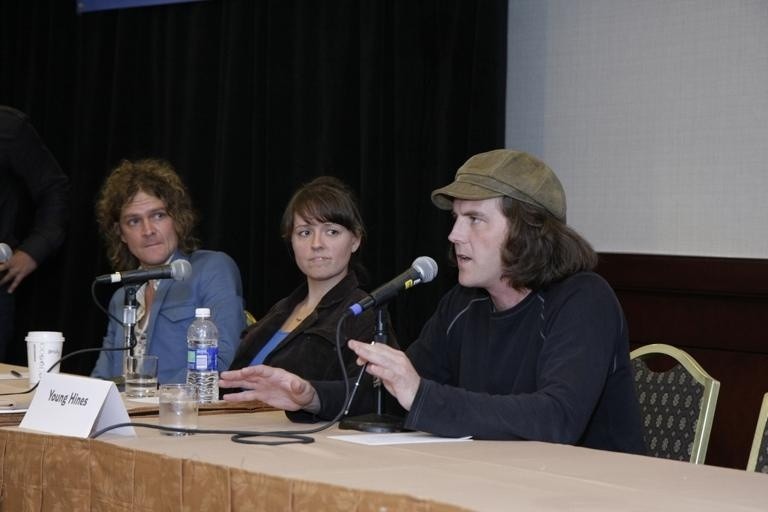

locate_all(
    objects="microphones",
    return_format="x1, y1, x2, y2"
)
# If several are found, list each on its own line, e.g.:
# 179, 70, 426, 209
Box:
95, 258, 191, 290
349, 255, 439, 316
0, 243, 13, 262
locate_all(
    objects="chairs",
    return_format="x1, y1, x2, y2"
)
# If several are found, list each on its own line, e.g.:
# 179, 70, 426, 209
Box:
746, 392, 768, 474
629, 342, 722, 466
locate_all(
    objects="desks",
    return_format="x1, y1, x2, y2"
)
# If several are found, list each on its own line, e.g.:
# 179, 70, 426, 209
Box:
0, 362, 768, 506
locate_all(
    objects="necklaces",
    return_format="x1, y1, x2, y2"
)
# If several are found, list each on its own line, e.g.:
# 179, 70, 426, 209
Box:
296, 296, 321, 321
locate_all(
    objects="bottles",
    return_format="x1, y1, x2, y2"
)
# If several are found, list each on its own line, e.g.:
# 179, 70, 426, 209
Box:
187, 308, 220, 403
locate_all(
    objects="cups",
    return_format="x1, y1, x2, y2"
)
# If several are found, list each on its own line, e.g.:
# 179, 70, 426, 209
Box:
24, 331, 65, 391
124, 355, 158, 398
160, 383, 198, 437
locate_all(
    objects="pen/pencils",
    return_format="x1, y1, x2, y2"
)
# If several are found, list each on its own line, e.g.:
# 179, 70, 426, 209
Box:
11, 370, 22, 378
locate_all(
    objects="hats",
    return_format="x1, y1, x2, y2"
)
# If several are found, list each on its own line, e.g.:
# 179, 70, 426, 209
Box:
430, 150, 568, 225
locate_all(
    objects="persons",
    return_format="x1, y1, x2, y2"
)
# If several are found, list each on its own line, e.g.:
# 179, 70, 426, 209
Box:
218, 149, 648, 455
0, 104, 77, 367
219, 177, 403, 401
90, 158, 247, 385
